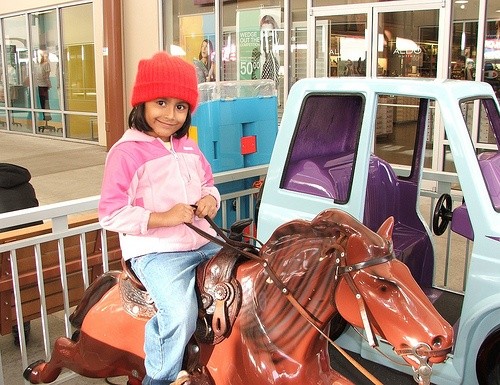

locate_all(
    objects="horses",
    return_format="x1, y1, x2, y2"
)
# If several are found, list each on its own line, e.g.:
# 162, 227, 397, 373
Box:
22, 207, 452, 385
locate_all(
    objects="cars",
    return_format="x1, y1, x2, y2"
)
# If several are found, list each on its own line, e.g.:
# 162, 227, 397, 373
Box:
249, 76, 500, 385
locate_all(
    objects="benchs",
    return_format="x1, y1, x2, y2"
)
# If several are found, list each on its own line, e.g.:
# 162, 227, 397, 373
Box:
283, 150, 433, 292
0, 210, 121, 338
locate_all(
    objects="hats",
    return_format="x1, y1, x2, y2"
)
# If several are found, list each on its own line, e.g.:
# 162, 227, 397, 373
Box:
131, 50, 198, 113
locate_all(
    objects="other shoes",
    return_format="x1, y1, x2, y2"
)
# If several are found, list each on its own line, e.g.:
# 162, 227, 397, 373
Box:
14, 328, 30, 345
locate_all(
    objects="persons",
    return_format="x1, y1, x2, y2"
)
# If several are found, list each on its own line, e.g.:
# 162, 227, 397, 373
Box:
97, 53, 225, 384
461, 58, 500, 100
250, 14, 280, 91
1, 52, 54, 121
191, 39, 217, 83
